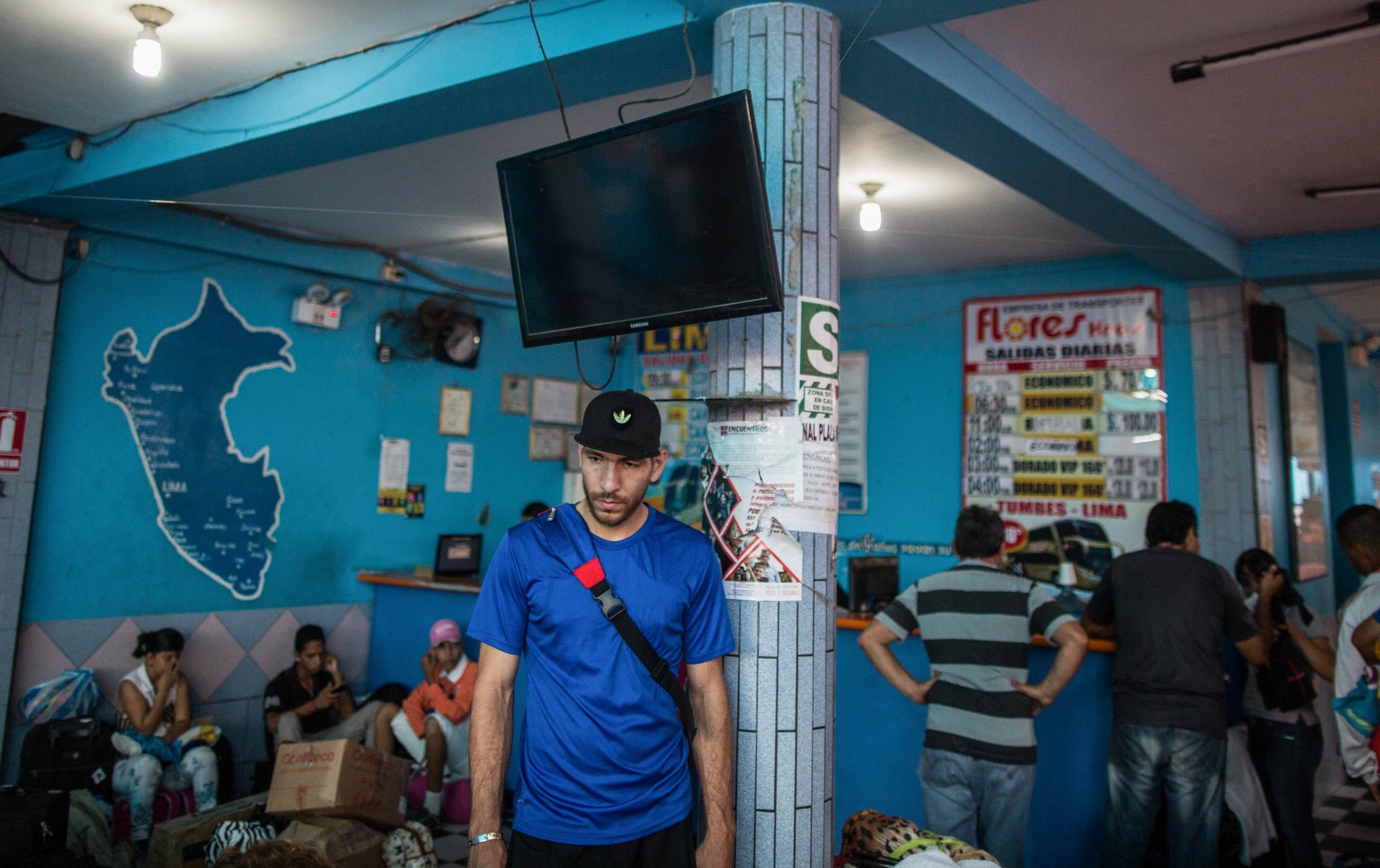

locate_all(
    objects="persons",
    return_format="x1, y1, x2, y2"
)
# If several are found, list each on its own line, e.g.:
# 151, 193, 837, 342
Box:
859, 502, 1379, 868
113, 622, 481, 868
466, 389, 736, 868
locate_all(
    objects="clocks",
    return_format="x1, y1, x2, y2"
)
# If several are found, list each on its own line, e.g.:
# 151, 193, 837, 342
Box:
434, 314, 482, 370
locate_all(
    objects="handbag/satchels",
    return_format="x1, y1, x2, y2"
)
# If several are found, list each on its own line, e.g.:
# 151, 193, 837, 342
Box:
17, 717, 114, 802
0, 782, 114, 868
1249, 570, 1317, 713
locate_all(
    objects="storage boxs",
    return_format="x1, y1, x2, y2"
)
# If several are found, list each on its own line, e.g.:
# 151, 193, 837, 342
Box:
265, 739, 409, 868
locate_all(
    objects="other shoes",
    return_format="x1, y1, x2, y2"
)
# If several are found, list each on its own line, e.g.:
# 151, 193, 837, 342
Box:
134, 839, 152, 857
413, 804, 449, 830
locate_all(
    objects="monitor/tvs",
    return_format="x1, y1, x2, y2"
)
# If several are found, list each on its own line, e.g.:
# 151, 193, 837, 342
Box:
846, 556, 900, 614
495, 90, 783, 348
435, 534, 482, 576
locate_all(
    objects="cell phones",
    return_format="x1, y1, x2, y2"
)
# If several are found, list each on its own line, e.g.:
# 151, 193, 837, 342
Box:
331, 684, 349, 694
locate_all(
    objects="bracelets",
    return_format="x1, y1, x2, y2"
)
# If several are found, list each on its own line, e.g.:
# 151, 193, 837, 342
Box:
313, 699, 319, 710
470, 831, 502, 847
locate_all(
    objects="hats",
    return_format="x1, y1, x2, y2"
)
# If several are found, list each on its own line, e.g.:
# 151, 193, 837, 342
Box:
132, 626, 185, 658
430, 620, 460, 649
575, 387, 663, 457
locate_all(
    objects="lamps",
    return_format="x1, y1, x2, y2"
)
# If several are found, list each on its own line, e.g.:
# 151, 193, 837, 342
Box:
291, 282, 351, 331
130, 5, 174, 77
859, 183, 882, 232
